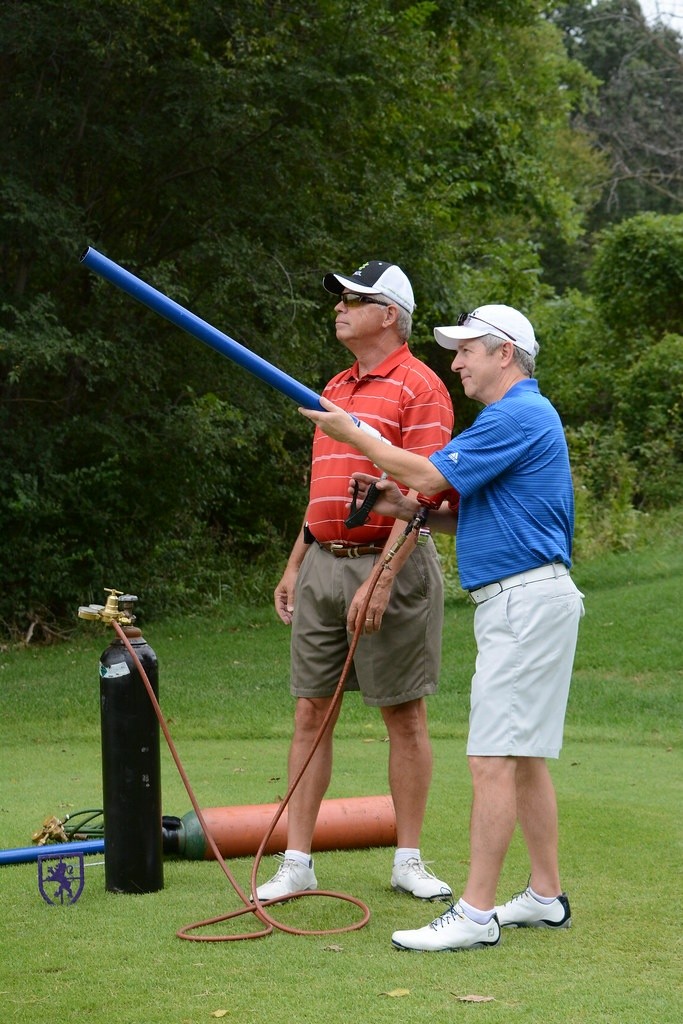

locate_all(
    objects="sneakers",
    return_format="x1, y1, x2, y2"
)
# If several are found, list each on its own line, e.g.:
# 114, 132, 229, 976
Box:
389, 902, 502, 951
247, 852, 319, 902
391, 857, 456, 902
493, 872, 572, 935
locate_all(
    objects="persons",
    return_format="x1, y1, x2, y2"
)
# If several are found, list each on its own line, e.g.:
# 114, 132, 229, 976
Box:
299, 304, 585, 953
250, 261, 453, 903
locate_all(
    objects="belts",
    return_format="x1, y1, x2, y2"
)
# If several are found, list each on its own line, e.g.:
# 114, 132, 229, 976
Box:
315, 541, 386, 557
469, 561, 570, 608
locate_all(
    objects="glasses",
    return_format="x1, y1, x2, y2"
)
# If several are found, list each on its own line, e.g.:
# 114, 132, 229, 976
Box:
336, 293, 391, 308
455, 312, 517, 341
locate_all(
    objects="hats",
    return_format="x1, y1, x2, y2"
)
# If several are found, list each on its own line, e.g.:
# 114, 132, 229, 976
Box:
434, 304, 537, 359
323, 260, 415, 313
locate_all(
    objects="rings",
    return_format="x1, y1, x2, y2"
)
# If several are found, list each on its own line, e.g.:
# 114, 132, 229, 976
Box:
365, 618, 373, 621
